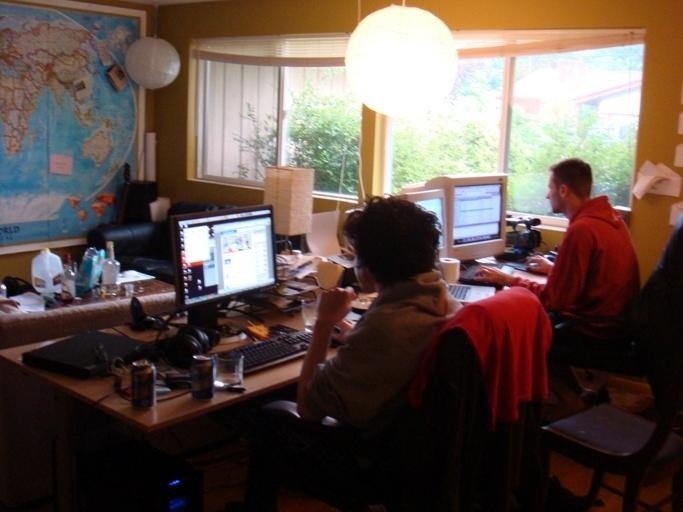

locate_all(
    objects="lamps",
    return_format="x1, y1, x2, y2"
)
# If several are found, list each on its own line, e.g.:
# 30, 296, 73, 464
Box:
262, 163, 315, 252
124, 5, 181, 90
344, 0, 453, 117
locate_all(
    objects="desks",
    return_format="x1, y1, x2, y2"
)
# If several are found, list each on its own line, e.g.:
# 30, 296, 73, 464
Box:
1, 259, 562, 511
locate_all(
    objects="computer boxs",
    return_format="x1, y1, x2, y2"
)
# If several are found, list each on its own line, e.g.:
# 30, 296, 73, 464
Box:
78, 444, 204, 512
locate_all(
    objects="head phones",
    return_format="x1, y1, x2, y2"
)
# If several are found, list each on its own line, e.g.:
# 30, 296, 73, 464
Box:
168, 325, 210, 367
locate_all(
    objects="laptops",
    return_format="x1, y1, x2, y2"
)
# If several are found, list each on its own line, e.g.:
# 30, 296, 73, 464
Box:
20, 330, 143, 380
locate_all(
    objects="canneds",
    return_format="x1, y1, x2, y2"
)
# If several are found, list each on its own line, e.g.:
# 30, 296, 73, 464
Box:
191, 353, 215, 400
130, 359, 156, 408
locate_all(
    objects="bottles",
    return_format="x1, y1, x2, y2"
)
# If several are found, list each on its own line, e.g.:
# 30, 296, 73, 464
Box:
101, 241, 120, 285
60, 253, 76, 297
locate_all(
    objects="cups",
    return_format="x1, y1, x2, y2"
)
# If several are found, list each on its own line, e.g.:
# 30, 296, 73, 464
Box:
211, 351, 244, 389
301, 298, 316, 332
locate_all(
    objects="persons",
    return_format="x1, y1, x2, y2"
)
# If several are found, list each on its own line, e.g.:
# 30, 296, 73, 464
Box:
218, 194, 465, 511
473, 158, 640, 414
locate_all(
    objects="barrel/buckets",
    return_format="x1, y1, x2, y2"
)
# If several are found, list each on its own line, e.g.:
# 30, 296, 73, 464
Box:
28, 247, 64, 295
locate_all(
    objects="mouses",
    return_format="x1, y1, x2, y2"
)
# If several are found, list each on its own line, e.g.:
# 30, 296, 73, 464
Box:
528, 262, 539, 267
333, 326, 341, 334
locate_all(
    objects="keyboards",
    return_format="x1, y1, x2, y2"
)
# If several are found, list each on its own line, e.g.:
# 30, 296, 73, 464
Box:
215, 330, 314, 376
459, 263, 514, 289
447, 285, 469, 301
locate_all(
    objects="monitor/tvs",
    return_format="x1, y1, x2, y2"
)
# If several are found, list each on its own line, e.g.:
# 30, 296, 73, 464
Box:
402, 173, 508, 261
394, 188, 448, 258
170, 204, 279, 344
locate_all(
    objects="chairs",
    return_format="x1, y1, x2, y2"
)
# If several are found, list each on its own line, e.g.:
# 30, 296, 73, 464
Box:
549, 215, 682, 408
88, 203, 246, 281
539, 370, 681, 512
245, 286, 551, 510
